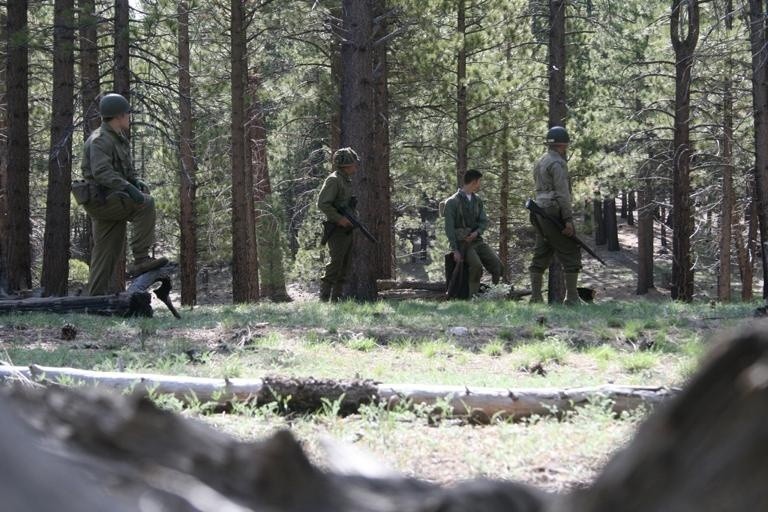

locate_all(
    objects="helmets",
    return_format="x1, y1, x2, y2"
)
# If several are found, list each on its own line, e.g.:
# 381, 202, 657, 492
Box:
100, 94, 130, 117
544, 126, 569, 144
333, 148, 360, 167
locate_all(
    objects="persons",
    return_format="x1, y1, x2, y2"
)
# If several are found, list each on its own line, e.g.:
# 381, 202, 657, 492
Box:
79, 93, 169, 298
316, 145, 362, 304
442, 168, 507, 302
526, 125, 593, 306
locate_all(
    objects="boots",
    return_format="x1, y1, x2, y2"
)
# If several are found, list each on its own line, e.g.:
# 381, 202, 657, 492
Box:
565, 272, 588, 307
530, 273, 543, 304
332, 283, 345, 304
319, 282, 330, 302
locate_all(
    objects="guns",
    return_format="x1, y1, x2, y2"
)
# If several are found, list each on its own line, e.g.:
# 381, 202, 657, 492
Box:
525, 198, 607, 265
321, 220, 335, 244
336, 197, 378, 243
446, 243, 465, 299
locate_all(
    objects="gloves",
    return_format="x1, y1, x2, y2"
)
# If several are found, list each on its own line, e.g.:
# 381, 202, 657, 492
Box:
125, 184, 144, 203
136, 181, 149, 194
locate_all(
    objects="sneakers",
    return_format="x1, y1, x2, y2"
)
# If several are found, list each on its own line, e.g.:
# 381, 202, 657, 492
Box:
87, 309, 114, 317
130, 256, 168, 277
